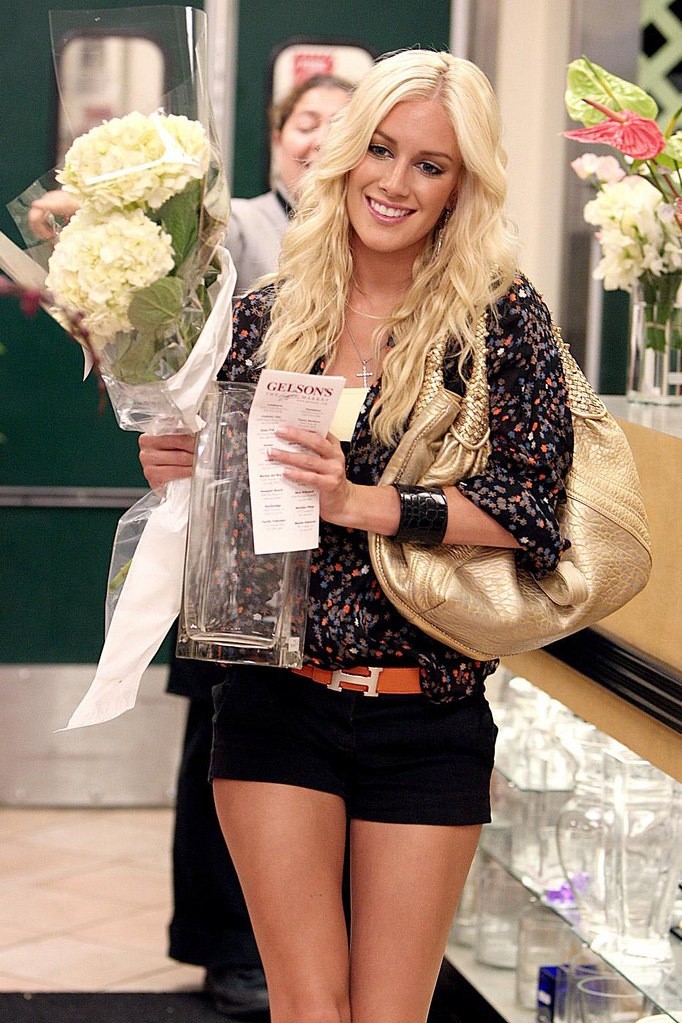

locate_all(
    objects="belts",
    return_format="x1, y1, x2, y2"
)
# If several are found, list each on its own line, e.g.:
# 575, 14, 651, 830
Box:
293, 667, 423, 697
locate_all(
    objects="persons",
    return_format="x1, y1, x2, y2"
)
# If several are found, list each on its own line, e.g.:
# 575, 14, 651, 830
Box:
28, 73, 357, 1017
138, 48, 576, 1022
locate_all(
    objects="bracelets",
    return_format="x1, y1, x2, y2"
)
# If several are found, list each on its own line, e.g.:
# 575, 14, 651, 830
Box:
387, 484, 448, 546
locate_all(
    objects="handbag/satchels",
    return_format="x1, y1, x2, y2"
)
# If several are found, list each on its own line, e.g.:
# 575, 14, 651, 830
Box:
368, 261, 655, 663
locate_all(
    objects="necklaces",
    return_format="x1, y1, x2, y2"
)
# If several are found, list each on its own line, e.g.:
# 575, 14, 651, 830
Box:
343, 310, 389, 388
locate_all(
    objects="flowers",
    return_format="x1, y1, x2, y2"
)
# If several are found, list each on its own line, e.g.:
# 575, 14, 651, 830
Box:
44, 110, 222, 394
562, 53, 682, 348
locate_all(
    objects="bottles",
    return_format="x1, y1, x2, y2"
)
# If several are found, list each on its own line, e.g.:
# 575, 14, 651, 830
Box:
486, 669, 679, 967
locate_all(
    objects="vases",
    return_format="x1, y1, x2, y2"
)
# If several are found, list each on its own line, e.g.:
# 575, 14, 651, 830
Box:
625, 272, 682, 406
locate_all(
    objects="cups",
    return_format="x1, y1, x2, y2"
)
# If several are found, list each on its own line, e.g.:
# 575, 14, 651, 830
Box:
446, 844, 646, 1023
175, 379, 309, 669
